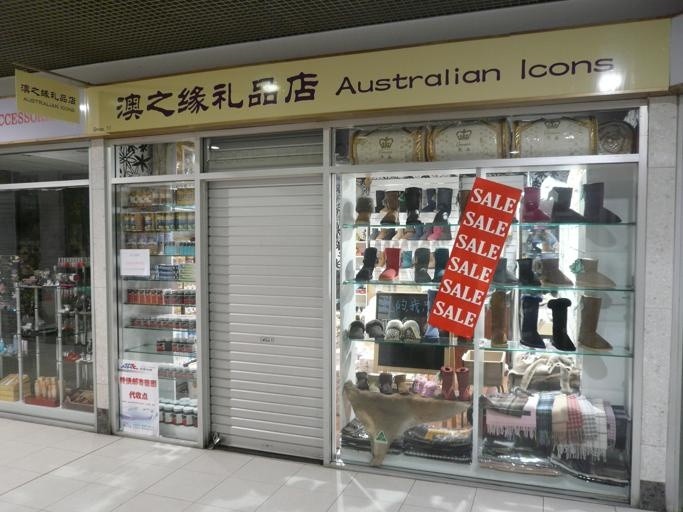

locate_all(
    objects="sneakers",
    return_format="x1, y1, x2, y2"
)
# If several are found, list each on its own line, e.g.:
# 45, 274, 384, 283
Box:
350, 319, 420, 341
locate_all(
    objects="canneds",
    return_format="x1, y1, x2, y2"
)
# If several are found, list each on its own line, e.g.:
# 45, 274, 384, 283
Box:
120, 213, 195, 232
127, 187, 195, 207
158, 398, 197, 426
156, 337, 195, 353
130, 316, 194, 329
164, 290, 196, 305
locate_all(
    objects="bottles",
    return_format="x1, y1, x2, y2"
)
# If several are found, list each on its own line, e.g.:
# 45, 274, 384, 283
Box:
163, 240, 193, 256
34, 376, 59, 400
120, 213, 196, 234
124, 288, 198, 306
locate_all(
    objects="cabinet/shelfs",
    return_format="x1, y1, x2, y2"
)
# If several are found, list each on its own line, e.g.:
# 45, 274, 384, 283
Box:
108, 180, 198, 443
330, 111, 641, 492
0, 256, 93, 418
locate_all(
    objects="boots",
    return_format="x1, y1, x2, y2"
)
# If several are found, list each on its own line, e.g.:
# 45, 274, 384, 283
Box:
355, 371, 438, 398
521, 182, 622, 222
355, 248, 449, 282
490, 257, 615, 351
355, 188, 471, 241
440, 366, 470, 401
424, 290, 440, 341
